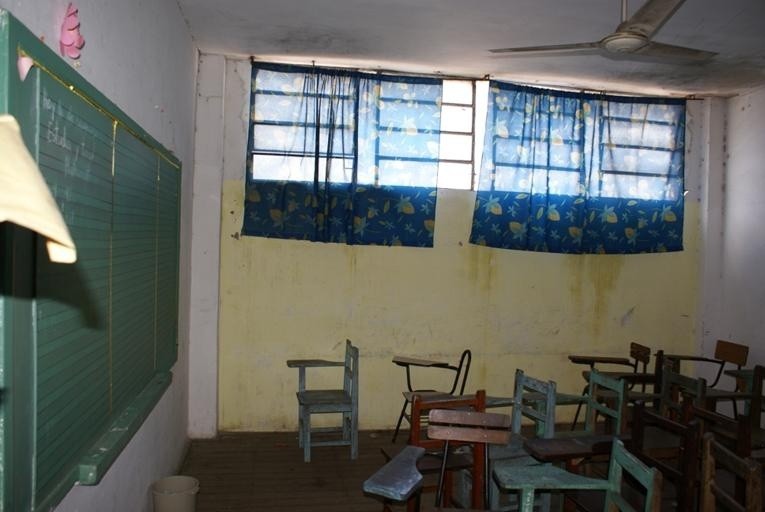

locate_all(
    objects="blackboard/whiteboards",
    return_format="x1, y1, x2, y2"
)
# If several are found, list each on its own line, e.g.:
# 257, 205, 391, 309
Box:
0, 7, 182, 512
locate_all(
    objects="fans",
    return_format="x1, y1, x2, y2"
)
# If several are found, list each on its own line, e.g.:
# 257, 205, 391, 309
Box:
488, 1, 719, 62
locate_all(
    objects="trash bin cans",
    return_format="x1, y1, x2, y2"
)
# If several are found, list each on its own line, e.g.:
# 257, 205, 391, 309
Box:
151, 475, 200, 512
